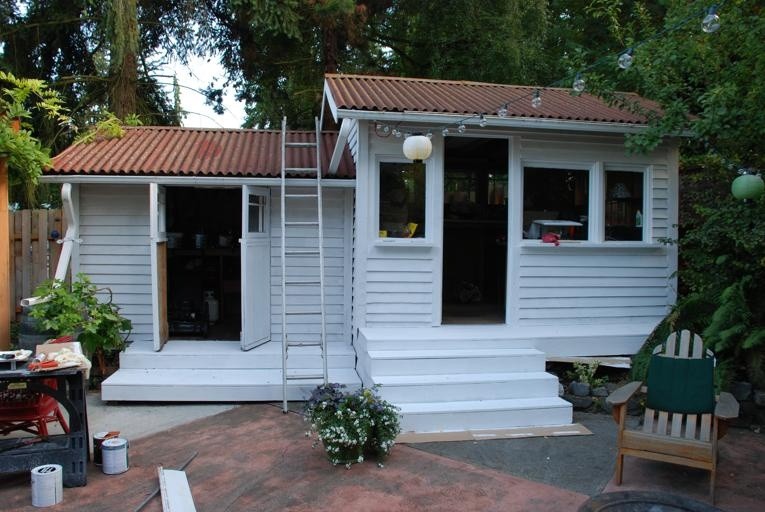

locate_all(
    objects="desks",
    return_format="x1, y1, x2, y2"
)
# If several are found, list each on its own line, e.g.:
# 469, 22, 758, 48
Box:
0, 350, 91, 488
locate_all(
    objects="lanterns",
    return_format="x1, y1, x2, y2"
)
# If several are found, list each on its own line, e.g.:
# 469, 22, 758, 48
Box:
732, 170, 764, 203
402, 134, 432, 163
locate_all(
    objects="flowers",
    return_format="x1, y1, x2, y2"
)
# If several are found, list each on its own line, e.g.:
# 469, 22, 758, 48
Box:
302, 383, 402, 469
571, 358, 603, 384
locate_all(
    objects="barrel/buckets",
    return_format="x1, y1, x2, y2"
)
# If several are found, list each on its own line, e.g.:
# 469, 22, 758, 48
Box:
31, 463, 64, 509
101, 438, 130, 475
93, 432, 119, 467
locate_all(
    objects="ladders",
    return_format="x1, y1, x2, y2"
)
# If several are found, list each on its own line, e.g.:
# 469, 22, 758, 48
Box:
281, 116, 328, 414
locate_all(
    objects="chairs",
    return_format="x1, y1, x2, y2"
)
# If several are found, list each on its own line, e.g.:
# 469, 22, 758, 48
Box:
1, 335, 76, 439
606, 329, 741, 508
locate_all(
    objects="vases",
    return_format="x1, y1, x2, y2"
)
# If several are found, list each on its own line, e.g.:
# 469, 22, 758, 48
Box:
572, 382, 591, 396
321, 440, 375, 463
